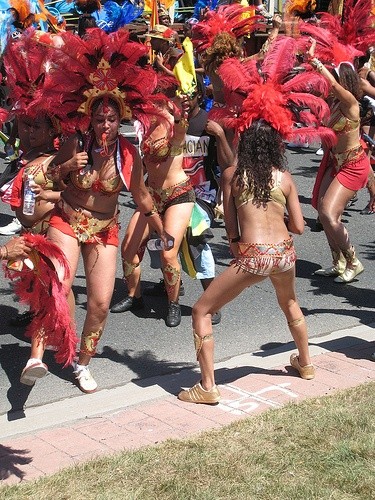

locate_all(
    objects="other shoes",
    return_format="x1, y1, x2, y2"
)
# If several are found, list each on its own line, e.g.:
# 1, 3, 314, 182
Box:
19, 357, 48, 386
0, 217, 22, 235
72, 364, 96, 393
144, 276, 188, 294
345, 193, 358, 206
210, 311, 221, 324
315, 148, 323, 155
359, 196, 375, 214
287, 139, 309, 148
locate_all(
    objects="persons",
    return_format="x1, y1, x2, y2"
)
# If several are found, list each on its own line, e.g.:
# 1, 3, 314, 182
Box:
108, 97, 196, 324
144, 74, 234, 322
12, 111, 75, 323
0, 16, 375, 235
20, 97, 174, 393
178, 120, 313, 404
0, 236, 31, 261
307, 37, 369, 283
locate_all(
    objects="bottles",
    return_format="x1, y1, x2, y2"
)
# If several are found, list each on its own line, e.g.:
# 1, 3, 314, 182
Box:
147, 239, 173, 250
23, 175, 36, 216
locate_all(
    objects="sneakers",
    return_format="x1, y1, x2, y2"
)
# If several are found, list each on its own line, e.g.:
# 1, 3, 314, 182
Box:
164, 300, 181, 327
177, 380, 220, 402
110, 295, 146, 311
290, 352, 314, 379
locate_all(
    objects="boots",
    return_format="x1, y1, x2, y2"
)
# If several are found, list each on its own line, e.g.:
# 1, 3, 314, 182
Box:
314, 247, 347, 275
332, 245, 364, 283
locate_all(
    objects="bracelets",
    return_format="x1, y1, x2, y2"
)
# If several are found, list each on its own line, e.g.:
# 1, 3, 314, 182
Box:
317, 64, 323, 70
4, 245, 8, 260
144, 205, 158, 217
313, 60, 320, 68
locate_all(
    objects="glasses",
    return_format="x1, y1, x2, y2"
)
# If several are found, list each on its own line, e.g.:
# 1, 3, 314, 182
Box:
187, 92, 198, 100
